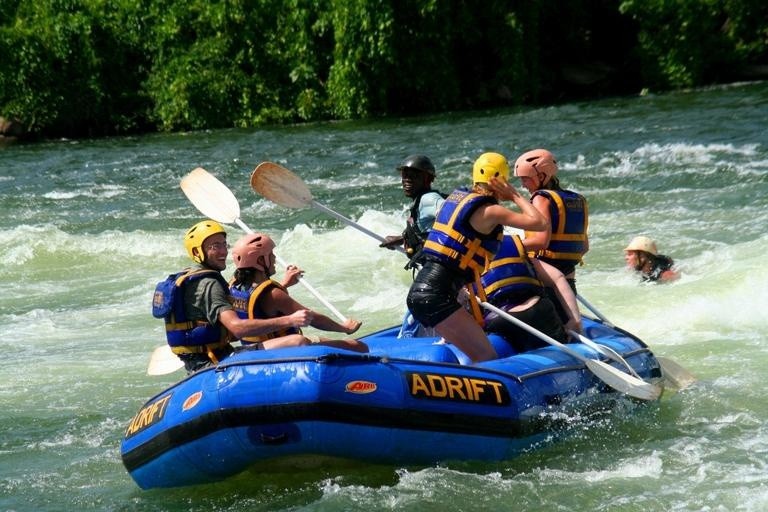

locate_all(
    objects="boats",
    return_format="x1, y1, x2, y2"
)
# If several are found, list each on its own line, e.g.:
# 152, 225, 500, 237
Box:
121, 314, 664, 489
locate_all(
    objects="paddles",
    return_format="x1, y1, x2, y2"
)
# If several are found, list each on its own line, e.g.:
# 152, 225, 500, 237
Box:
180, 167, 350, 324
250, 162, 412, 258
576, 293, 696, 389
563, 328, 643, 381
465, 288, 662, 400
147, 344, 186, 376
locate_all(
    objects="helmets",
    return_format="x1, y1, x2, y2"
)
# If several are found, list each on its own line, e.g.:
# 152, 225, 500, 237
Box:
231, 234, 276, 278
624, 236, 659, 269
513, 148, 558, 190
472, 151, 509, 187
182, 217, 227, 267
396, 154, 436, 191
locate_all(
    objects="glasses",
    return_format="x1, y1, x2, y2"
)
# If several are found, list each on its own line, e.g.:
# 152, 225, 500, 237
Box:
211, 241, 227, 249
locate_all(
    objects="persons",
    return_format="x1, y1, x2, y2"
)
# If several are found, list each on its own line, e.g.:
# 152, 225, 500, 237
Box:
380, 153, 445, 256
153, 220, 314, 370
406, 153, 549, 362
513, 148, 588, 300
228, 232, 367, 352
479, 237, 581, 351
623, 236, 674, 283
